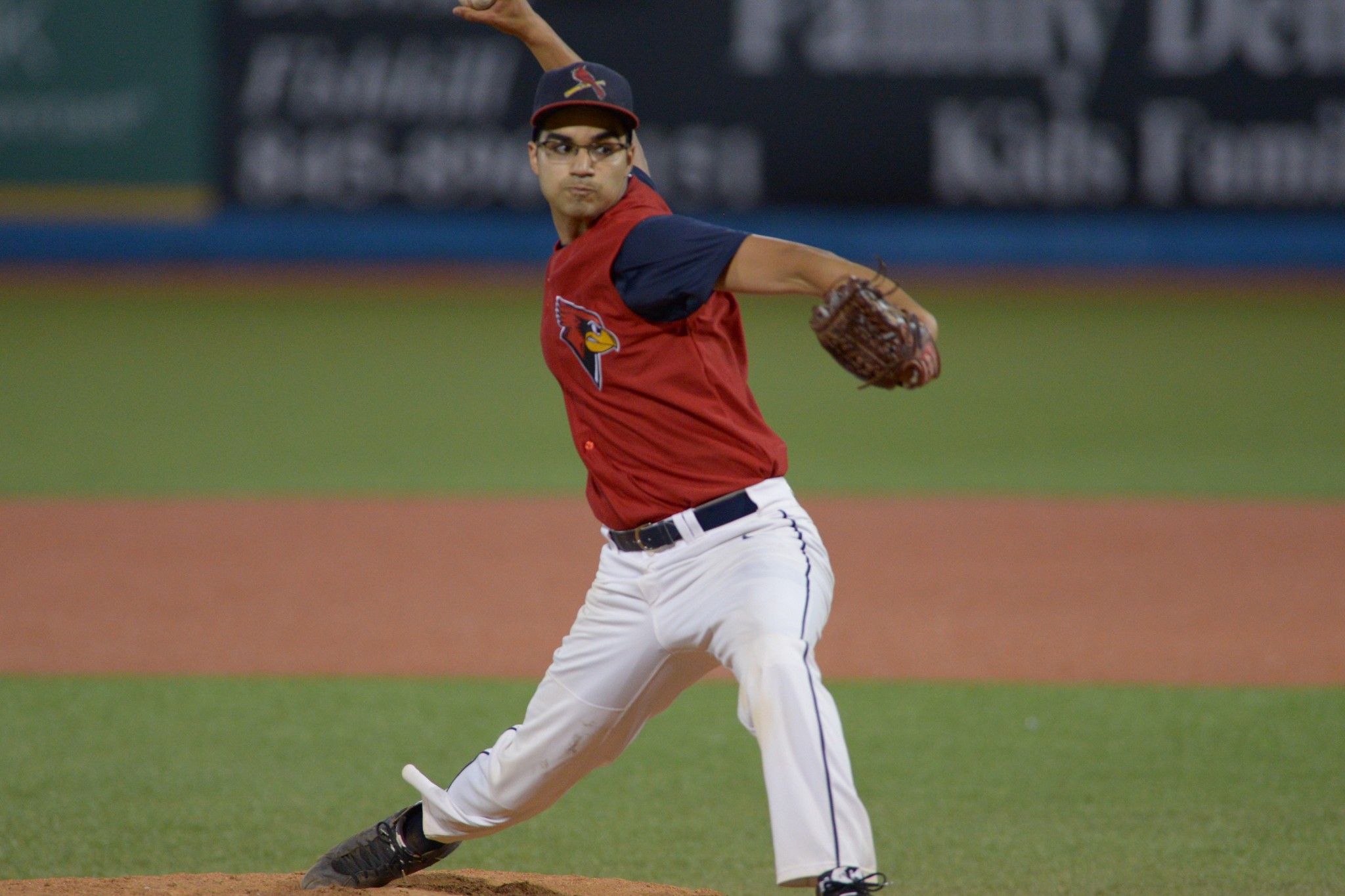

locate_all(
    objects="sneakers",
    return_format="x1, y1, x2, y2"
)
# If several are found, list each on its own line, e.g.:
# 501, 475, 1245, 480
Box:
815, 864, 887, 896
304, 800, 463, 890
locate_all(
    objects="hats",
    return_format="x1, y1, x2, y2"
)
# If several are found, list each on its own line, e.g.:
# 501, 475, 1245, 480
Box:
530, 61, 639, 133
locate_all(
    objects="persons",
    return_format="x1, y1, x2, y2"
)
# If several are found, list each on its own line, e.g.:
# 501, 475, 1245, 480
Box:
298, 0, 936, 896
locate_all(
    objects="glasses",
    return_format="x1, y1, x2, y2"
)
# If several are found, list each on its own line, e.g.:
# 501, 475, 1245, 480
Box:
537, 140, 629, 166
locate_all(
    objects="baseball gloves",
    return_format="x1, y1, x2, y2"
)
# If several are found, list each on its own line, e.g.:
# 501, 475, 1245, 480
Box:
808, 273, 942, 391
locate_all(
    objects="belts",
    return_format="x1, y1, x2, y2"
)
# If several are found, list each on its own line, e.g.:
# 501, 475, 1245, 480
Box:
610, 491, 756, 554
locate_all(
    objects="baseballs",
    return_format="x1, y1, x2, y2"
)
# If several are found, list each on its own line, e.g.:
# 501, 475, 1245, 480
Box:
458, 0, 497, 11
